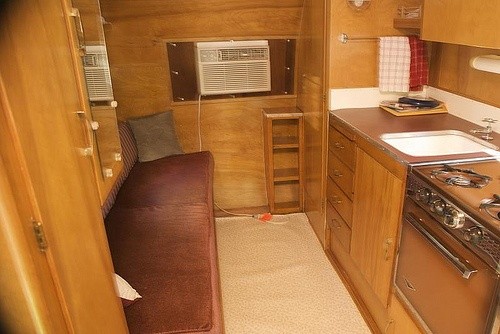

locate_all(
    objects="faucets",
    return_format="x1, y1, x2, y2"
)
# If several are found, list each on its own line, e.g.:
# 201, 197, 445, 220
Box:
470, 118, 498, 140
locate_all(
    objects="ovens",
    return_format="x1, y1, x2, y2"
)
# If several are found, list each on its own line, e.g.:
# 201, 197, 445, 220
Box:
388, 196, 500, 334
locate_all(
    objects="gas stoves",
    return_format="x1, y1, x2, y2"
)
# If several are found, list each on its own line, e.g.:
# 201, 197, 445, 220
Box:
404, 154, 500, 276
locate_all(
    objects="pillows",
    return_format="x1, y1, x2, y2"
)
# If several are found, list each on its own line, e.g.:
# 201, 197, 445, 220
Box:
127, 111, 184, 164
114, 274, 141, 309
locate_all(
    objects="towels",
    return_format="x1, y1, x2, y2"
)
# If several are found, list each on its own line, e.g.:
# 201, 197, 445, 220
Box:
409, 34, 428, 92
379, 36, 411, 93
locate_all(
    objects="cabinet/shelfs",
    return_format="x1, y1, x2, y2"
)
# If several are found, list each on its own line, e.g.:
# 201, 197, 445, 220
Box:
0, 0, 500, 334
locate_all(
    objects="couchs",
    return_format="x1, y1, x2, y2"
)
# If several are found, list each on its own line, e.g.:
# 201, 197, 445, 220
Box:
101, 120, 224, 334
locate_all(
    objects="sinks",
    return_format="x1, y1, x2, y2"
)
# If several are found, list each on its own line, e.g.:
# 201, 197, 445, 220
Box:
378, 130, 494, 156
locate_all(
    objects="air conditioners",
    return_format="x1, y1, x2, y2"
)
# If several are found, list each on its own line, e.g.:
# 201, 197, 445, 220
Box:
82, 45, 114, 102
194, 40, 272, 95
